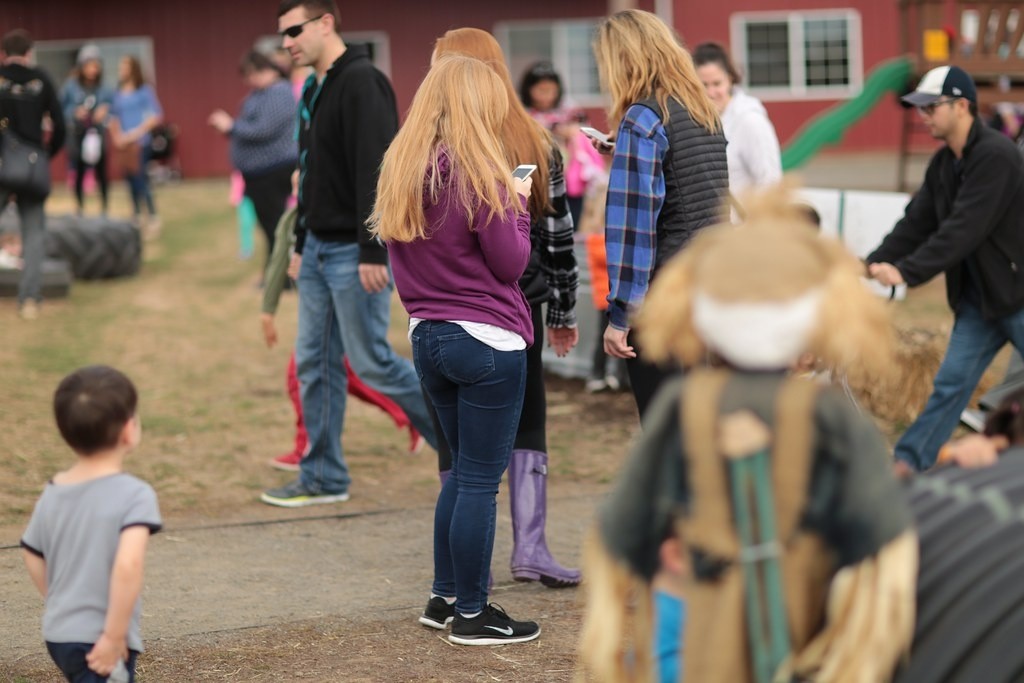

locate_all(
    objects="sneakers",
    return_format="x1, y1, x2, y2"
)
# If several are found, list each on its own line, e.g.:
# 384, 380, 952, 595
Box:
448, 602, 541, 646
418, 595, 458, 628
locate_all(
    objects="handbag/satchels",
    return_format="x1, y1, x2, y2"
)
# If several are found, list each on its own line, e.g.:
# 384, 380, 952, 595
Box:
76, 109, 110, 171
1, 127, 52, 201
105, 141, 140, 179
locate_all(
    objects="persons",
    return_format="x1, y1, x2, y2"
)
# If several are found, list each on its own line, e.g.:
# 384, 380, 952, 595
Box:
59, 45, 115, 216
691, 42, 783, 229
517, 62, 606, 230
20, 364, 162, 683
427, 27, 582, 586
107, 53, 166, 241
858, 66, 1024, 479
369, 53, 541, 646
210, 0, 440, 508
0, 28, 68, 323
593, 9, 730, 430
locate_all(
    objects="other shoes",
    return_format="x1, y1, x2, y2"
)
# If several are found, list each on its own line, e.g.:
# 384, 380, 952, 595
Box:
586, 377, 620, 395
259, 479, 351, 508
20, 296, 40, 323
271, 452, 304, 471
141, 214, 162, 240
408, 425, 425, 456
959, 407, 991, 429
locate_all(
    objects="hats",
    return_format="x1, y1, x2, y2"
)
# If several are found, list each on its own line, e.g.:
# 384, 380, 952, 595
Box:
900, 66, 976, 111
518, 60, 565, 107
237, 35, 295, 79
77, 44, 104, 67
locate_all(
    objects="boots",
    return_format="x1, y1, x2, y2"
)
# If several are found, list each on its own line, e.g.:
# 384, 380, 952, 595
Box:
508, 448, 586, 587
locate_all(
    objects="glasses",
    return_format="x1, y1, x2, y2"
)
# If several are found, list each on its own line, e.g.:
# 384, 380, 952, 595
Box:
278, 15, 321, 38
921, 99, 957, 115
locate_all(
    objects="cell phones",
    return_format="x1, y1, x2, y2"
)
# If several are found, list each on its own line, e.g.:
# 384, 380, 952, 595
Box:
511, 165, 537, 183
580, 127, 616, 148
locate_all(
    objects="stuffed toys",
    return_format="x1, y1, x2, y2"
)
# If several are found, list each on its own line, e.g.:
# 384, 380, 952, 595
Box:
573, 182, 920, 682
